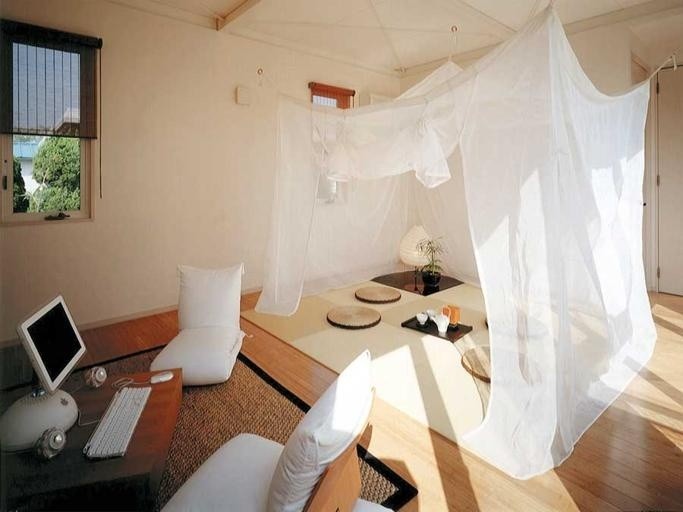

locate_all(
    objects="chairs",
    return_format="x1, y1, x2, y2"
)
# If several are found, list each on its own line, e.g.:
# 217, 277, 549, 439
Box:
161, 387, 375, 511
147, 264, 247, 384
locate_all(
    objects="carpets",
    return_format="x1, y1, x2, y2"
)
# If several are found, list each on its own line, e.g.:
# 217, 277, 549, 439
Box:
242, 267, 630, 480
0, 345, 418, 512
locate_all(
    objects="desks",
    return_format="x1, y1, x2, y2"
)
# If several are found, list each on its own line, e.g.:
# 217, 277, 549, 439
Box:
0, 368, 182, 512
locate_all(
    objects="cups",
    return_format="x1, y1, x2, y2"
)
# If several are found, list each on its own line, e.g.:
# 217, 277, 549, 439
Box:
426, 309, 436, 320
416, 314, 428, 324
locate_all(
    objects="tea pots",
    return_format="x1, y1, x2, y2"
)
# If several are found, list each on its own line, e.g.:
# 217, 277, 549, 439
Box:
429, 314, 450, 333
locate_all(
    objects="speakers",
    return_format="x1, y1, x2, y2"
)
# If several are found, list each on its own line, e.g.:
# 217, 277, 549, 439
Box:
83, 367, 107, 389
33, 427, 67, 461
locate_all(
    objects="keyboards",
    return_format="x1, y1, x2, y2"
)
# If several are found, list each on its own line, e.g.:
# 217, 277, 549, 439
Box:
82, 386, 151, 459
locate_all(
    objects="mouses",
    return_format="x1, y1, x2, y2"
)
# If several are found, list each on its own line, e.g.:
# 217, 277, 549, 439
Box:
151, 371, 174, 384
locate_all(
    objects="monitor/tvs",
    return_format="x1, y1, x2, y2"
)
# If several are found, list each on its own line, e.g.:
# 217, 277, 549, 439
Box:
15, 293, 87, 396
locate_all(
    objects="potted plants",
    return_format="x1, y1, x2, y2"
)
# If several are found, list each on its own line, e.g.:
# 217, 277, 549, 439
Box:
416, 235, 448, 285
